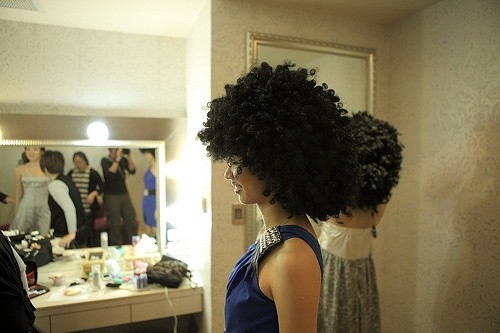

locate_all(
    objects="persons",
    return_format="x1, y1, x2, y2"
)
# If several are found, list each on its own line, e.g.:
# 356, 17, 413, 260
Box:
198, 64, 352, 333
0, 230, 37, 333
0, 144, 159, 251
316, 112, 404, 333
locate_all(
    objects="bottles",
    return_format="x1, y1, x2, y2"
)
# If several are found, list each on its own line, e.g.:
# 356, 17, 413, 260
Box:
91, 264, 101, 291
101, 231, 108, 252
137, 270, 147, 290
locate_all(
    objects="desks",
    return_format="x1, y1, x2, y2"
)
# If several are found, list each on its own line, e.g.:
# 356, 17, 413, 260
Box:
30, 276, 204, 333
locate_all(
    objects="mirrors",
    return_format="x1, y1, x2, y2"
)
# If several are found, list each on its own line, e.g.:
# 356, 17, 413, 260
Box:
1, 140, 167, 272
245, 31, 377, 255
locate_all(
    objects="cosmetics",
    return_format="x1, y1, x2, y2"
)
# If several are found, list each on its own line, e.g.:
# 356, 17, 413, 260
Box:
136, 272, 148, 289
93, 267, 102, 288
135, 235, 140, 245
99, 231, 109, 250
132, 235, 135, 245
